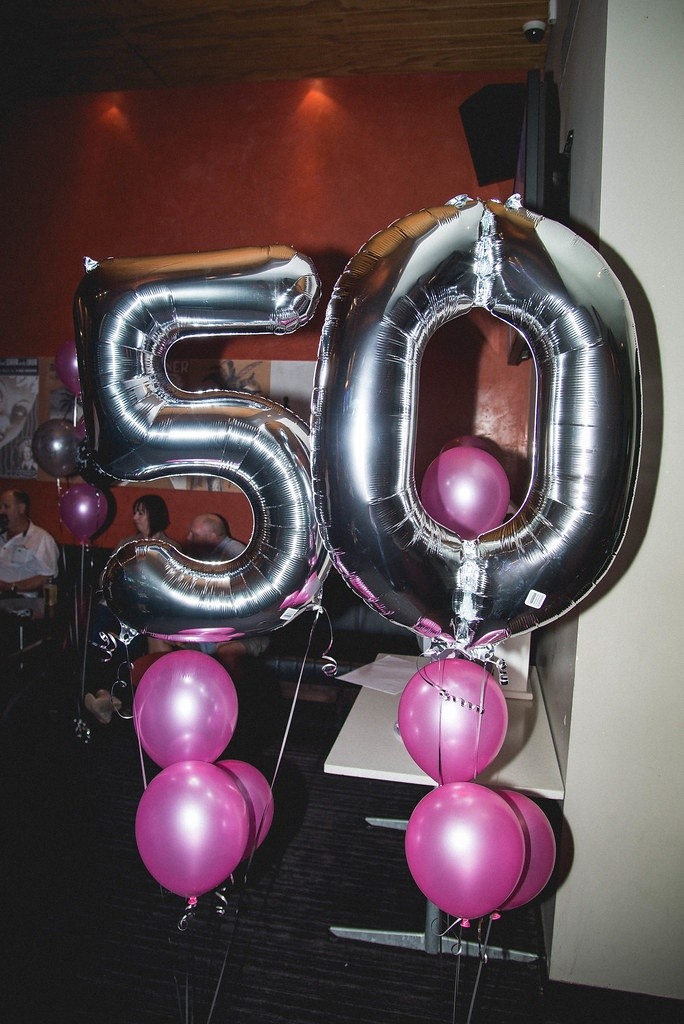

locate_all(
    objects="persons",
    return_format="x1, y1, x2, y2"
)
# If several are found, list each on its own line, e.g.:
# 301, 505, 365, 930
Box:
0, 489, 58, 598
77, 494, 271, 726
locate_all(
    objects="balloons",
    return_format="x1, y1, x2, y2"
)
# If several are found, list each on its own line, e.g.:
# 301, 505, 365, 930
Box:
59, 482, 109, 542
398, 658, 509, 784
421, 436, 512, 541
134, 760, 274, 905
31, 418, 83, 479
55, 323, 81, 404
131, 650, 239, 771
404, 784, 557, 927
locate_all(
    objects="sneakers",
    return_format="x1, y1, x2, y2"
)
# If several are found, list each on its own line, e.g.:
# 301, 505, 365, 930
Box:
83, 691, 122, 725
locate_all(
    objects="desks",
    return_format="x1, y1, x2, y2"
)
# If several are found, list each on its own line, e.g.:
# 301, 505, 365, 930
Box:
323, 654, 564, 962
